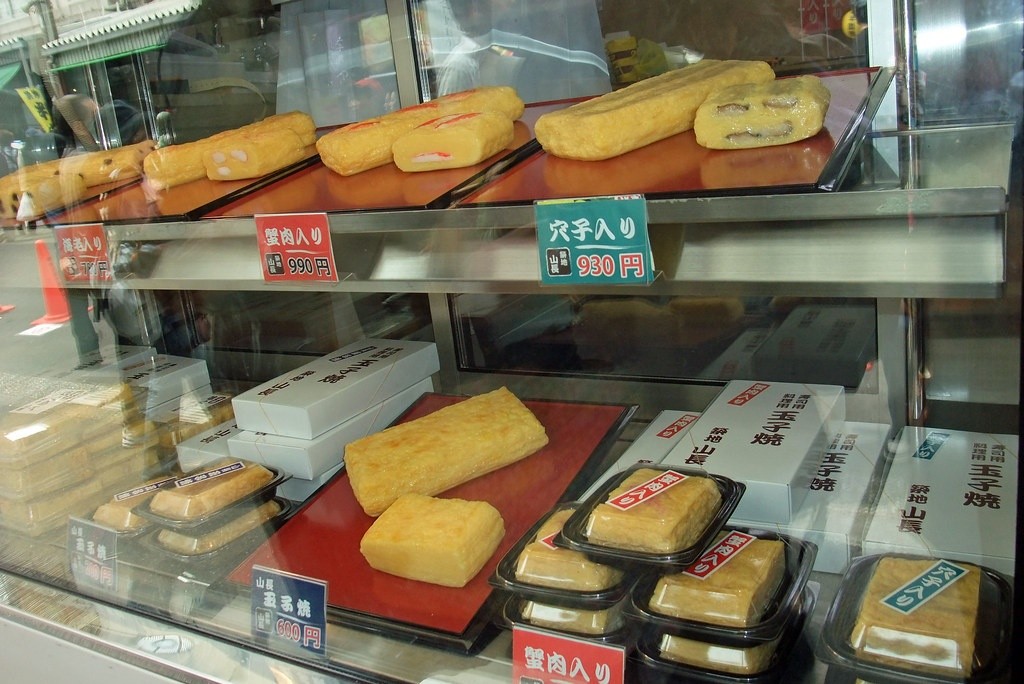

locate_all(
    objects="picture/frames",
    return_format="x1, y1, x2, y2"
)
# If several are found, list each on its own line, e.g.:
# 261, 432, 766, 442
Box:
0, 176, 144, 231
211, 391, 639, 656
196, 96, 600, 223
452, 65, 899, 209
25, 121, 359, 231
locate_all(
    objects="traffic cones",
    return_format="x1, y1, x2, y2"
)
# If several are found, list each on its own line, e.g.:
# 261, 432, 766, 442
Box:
30, 240, 95, 325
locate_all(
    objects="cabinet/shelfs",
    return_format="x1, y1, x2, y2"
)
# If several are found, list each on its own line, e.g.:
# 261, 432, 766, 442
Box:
0, 0, 1024, 684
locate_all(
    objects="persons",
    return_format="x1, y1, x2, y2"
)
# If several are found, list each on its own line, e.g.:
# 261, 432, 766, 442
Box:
1, 1, 884, 684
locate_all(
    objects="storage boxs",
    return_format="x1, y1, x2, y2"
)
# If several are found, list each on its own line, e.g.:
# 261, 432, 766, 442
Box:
576, 410, 702, 504
143, 386, 213, 419
37, 343, 159, 380
660, 380, 845, 525
232, 339, 441, 439
227, 378, 434, 480
106, 354, 211, 411
725, 421, 892, 574
862, 426, 1019, 587
175, 418, 243, 474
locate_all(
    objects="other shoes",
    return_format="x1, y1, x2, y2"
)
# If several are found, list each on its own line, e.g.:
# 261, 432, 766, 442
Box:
122, 429, 149, 448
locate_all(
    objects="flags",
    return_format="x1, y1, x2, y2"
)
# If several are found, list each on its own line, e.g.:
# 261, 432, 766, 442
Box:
15, 87, 52, 139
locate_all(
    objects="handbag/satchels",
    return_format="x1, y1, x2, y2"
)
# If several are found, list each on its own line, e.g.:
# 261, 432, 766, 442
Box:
16, 191, 42, 222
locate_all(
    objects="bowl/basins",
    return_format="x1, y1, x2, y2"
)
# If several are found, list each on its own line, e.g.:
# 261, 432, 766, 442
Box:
485, 461, 1014, 684
87, 456, 296, 569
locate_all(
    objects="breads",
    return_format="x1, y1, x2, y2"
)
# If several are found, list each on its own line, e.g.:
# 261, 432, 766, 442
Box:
0, 370, 983, 684
0, 58, 831, 218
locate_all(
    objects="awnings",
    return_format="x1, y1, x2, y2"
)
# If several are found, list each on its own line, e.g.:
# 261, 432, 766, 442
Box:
0, 60, 20, 89
41, 0, 202, 73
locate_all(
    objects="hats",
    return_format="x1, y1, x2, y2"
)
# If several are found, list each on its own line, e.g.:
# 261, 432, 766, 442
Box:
92, 99, 148, 148
